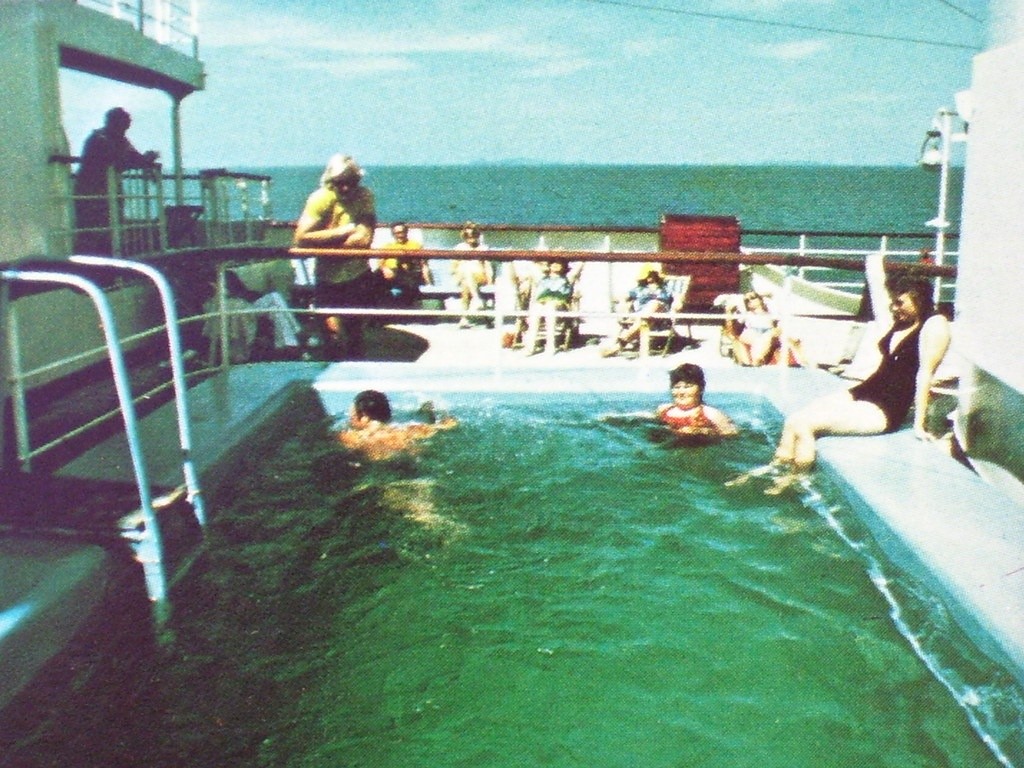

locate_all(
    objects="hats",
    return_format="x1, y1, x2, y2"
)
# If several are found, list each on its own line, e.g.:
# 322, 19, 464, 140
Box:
319, 151, 366, 186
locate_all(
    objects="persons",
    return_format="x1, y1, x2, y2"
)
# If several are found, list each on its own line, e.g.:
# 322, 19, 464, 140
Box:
727, 291, 781, 363
378, 220, 432, 300
524, 257, 583, 355
657, 363, 733, 436
600, 267, 673, 359
222, 264, 319, 360
72, 106, 160, 259
339, 391, 454, 444
446, 219, 494, 329
294, 152, 394, 350
779, 267, 950, 463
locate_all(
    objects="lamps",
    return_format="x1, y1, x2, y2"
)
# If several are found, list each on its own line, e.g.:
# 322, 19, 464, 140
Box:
917, 122, 950, 175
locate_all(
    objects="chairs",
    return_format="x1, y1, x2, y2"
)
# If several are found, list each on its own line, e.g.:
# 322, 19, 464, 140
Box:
604, 268, 695, 356
113, 205, 204, 255
498, 262, 590, 358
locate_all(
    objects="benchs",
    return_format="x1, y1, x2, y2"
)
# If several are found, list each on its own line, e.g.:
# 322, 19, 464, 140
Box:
383, 285, 500, 300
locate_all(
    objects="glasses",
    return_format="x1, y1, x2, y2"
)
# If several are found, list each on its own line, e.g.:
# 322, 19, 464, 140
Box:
329, 178, 361, 187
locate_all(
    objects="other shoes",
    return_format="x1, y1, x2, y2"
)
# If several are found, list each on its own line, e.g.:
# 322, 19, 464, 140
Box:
467, 296, 486, 310
458, 318, 470, 329
